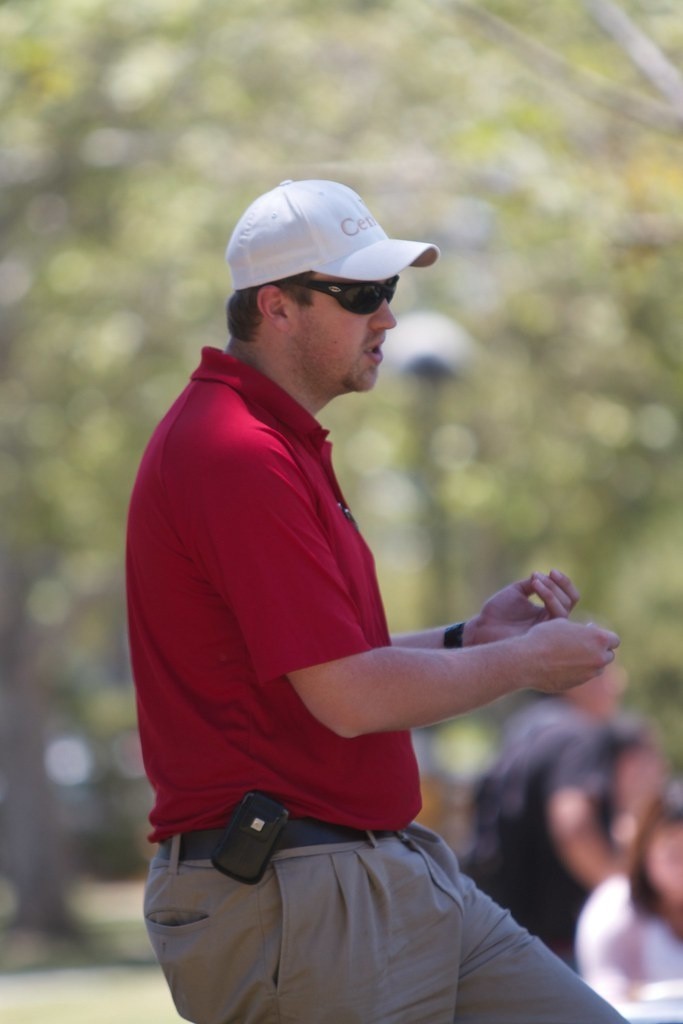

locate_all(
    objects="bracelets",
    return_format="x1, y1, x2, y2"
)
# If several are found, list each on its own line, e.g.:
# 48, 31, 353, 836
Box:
445, 623, 466, 649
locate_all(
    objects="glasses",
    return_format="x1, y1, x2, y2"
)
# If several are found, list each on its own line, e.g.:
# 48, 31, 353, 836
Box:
278, 275, 398, 314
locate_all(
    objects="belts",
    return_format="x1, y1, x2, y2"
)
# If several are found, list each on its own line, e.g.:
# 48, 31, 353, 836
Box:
158, 817, 398, 859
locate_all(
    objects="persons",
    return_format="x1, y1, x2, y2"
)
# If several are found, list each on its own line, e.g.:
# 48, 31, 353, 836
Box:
462, 655, 683, 1024
120, 180, 638, 1024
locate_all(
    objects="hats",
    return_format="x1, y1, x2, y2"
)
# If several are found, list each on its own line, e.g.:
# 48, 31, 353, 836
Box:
224, 180, 441, 292
